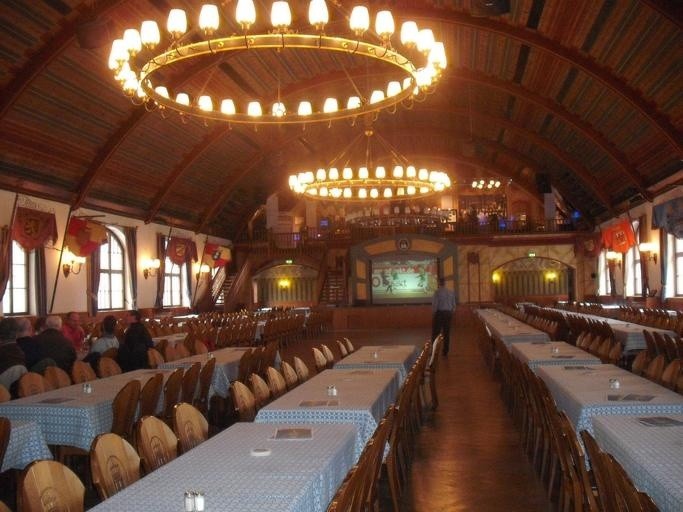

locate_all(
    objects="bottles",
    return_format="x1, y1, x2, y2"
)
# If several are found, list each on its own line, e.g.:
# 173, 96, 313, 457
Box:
184, 490, 194, 512
327, 384, 335, 396
195, 490, 204, 511
83, 383, 91, 394
608, 378, 620, 390
207, 352, 213, 359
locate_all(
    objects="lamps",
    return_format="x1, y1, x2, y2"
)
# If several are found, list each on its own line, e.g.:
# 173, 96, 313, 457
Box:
107, 1, 449, 129
61, 246, 86, 278
288, 118, 452, 207
470, 174, 513, 193
604, 251, 619, 264
638, 241, 659, 263
143, 259, 161, 280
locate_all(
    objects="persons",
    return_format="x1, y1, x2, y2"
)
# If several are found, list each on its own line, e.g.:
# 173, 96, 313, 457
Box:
14, 317, 57, 377
0, 317, 32, 400
38, 315, 78, 377
61, 312, 85, 354
119, 310, 153, 373
427, 277, 456, 357
81, 315, 124, 377
